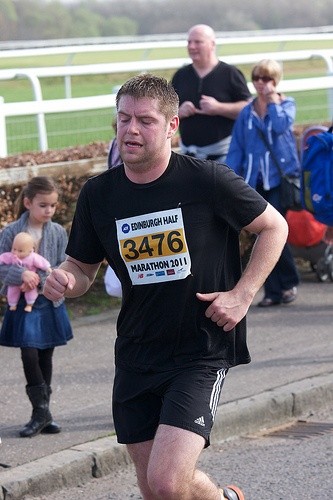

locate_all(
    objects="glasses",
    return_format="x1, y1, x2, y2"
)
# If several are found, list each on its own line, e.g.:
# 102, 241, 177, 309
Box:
251, 75, 274, 83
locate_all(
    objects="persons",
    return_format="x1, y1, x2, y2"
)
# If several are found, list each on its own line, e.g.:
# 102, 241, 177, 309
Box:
0, 177, 74, 437
170, 24, 253, 164
225, 59, 301, 307
0, 232, 53, 312
42, 73, 290, 500
107, 112, 124, 168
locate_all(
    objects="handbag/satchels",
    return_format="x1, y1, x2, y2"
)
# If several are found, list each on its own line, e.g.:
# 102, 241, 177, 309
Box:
282, 175, 302, 211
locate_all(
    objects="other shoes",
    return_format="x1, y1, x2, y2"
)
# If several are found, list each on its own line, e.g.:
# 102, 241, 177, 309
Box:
220, 485, 244, 500
280, 286, 297, 304
257, 297, 280, 308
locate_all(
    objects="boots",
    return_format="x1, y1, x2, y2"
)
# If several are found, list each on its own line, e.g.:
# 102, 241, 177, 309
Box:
18, 383, 62, 438
